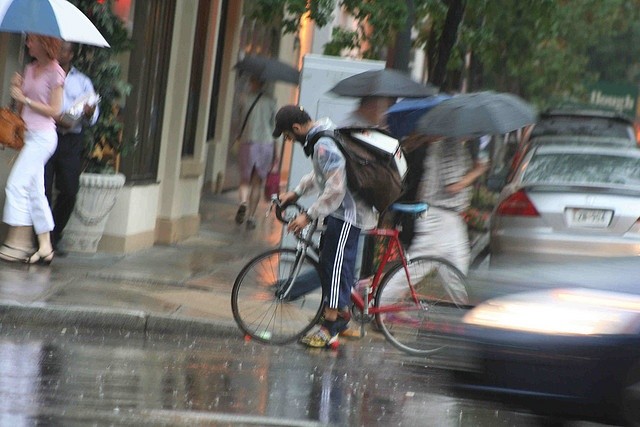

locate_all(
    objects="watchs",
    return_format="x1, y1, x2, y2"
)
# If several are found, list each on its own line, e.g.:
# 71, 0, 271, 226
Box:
305, 211, 313, 222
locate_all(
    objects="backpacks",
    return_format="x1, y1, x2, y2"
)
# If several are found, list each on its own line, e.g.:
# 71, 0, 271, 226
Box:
303, 127, 410, 213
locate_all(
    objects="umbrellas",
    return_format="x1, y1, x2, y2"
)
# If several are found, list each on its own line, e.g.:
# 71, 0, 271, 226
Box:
229, 54, 300, 86
381, 96, 450, 139
328, 68, 440, 97
412, 89, 539, 138
0, 0, 111, 118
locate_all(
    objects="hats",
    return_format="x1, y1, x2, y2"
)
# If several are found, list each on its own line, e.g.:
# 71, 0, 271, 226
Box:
272, 105, 303, 137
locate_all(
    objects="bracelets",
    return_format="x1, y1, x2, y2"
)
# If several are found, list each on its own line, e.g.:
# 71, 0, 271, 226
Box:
22, 97, 32, 107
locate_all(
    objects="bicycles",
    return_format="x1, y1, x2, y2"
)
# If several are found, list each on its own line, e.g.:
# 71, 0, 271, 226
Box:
231, 193, 475, 356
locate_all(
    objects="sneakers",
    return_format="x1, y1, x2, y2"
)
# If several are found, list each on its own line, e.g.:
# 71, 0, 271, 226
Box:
298, 327, 339, 348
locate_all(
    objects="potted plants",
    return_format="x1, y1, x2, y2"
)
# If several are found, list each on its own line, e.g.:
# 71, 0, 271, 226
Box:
54, 0, 137, 255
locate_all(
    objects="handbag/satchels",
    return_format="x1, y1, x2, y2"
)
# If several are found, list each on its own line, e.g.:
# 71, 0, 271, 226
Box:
1, 97, 27, 151
230, 92, 264, 157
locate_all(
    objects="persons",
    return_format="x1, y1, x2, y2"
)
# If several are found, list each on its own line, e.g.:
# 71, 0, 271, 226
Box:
380, 128, 445, 325
337, 96, 390, 339
378, 137, 493, 329
1, 31, 68, 267
34, 39, 100, 268
272, 105, 381, 349
233, 73, 285, 232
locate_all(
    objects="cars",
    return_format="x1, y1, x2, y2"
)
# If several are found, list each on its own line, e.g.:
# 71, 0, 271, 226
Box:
508, 135, 640, 181
404, 282, 639, 425
491, 144, 640, 267
519, 107, 637, 158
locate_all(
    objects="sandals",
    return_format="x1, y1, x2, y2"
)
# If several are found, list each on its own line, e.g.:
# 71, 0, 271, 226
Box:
236, 204, 247, 224
19, 250, 53, 266
245, 220, 256, 230
